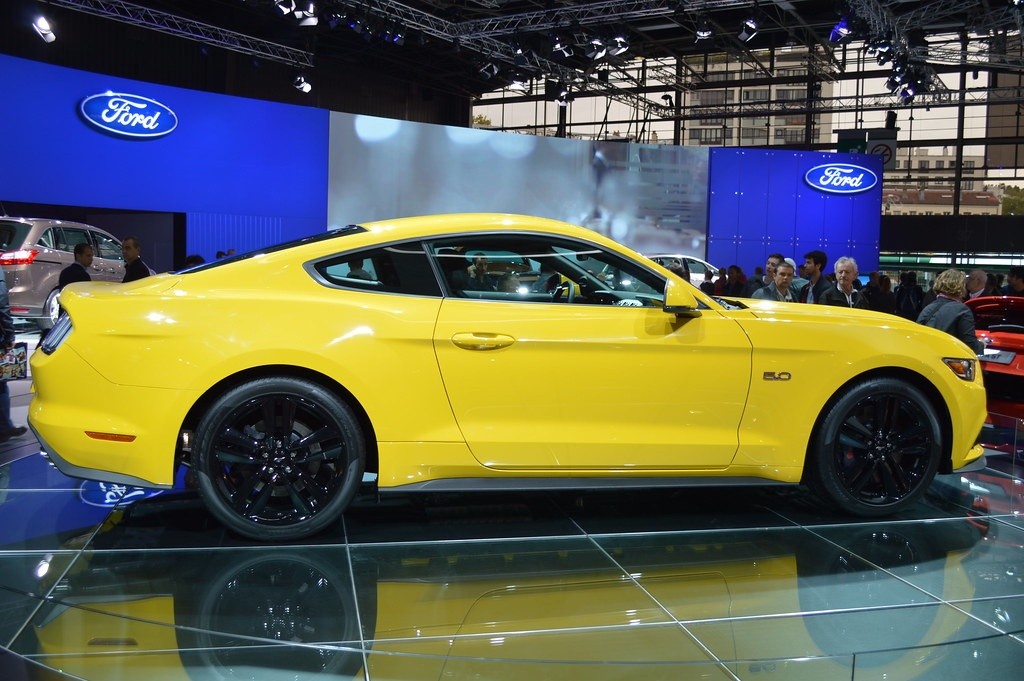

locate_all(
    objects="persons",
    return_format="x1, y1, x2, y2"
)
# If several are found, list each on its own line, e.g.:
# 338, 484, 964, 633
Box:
962, 265, 1024, 304
59, 243, 93, 318
916, 269, 987, 356
0, 266, 28, 443
467, 253, 497, 291
183, 255, 204, 270
216, 249, 235, 260
346, 257, 372, 281
751, 254, 810, 303
587, 269, 628, 292
818, 257, 860, 308
853, 272, 894, 314
894, 272, 925, 323
799, 251, 833, 304
700, 265, 763, 298
122, 237, 150, 284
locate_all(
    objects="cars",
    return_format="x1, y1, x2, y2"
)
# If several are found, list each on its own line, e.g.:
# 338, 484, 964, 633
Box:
24, 502, 998, 681
960, 459, 1024, 549
26, 214, 987, 542
962, 297, 1024, 453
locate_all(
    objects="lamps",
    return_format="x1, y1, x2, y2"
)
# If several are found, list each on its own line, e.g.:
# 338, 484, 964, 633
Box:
31, 0, 58, 43
324, 2, 409, 45
548, 22, 630, 60
292, 66, 314, 94
693, 6, 714, 43
736, 2, 760, 42
273, 0, 318, 27
830, 0, 926, 106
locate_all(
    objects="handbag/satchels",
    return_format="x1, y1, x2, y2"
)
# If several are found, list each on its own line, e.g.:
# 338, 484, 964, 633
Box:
0, 342, 28, 382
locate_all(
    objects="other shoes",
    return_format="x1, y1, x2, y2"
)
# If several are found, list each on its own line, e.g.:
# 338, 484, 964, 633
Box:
0, 426, 27, 442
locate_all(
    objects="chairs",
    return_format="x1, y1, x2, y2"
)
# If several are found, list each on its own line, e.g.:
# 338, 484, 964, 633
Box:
392, 246, 441, 292
369, 250, 409, 294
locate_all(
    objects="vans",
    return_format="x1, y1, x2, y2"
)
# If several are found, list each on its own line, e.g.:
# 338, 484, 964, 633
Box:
0, 216, 154, 330
